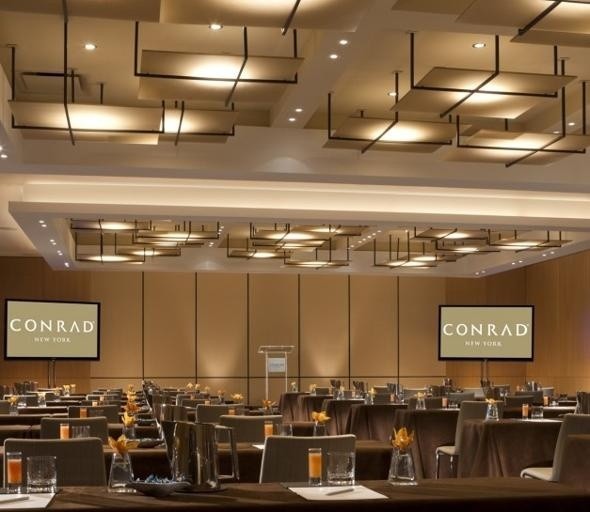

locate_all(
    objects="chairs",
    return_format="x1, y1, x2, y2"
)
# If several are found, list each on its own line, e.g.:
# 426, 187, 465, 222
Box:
0, 378, 590, 511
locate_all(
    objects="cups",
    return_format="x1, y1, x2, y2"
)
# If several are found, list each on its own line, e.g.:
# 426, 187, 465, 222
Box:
521, 403, 544, 420
274, 423, 293, 436
24, 455, 58, 494
326, 451, 357, 487
263, 420, 273, 438
59, 423, 69, 440
79, 395, 105, 419
190, 393, 237, 417
71, 425, 91, 439
307, 447, 322, 487
6, 451, 24, 494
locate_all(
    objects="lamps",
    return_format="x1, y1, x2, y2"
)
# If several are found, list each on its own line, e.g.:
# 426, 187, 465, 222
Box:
389, 31, 577, 120
440, 80, 588, 167
131, 23, 305, 114
8, 17, 163, 146
319, 71, 470, 159
152, 102, 237, 155
67, 216, 574, 271
